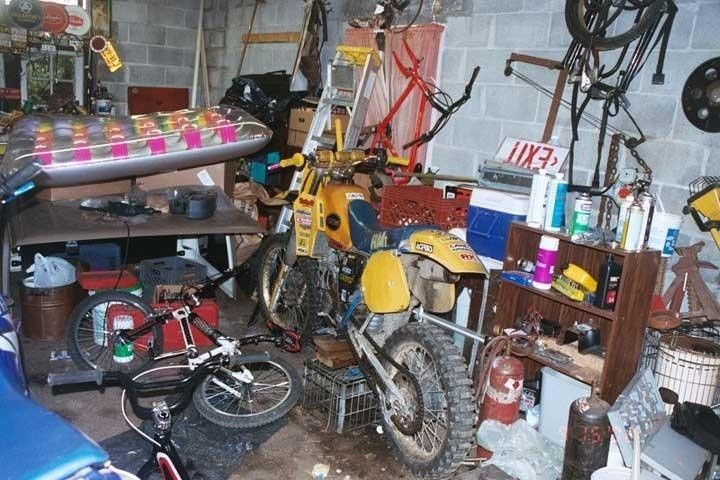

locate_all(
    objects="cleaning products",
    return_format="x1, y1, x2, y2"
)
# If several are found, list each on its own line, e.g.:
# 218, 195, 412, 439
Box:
544, 171, 567, 234
525, 168, 551, 230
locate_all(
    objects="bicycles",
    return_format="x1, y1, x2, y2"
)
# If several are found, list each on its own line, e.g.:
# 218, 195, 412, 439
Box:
46, 350, 273, 480
64, 262, 304, 429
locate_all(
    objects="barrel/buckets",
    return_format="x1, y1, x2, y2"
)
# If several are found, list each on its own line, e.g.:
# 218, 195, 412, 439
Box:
648, 210, 684, 259
654, 329, 720, 418
20, 275, 89, 341
87, 280, 144, 347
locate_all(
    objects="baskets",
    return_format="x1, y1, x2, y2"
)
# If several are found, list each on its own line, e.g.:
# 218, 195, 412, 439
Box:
380, 184, 469, 232
139, 256, 207, 292
301, 357, 378, 434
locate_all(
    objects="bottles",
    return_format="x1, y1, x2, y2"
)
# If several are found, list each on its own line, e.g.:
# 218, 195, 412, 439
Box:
570, 192, 593, 240
617, 191, 656, 254
456, 286, 472, 326
532, 235, 560, 289
527, 169, 569, 233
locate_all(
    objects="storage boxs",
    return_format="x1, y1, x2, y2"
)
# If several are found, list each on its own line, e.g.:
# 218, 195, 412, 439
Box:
457, 183, 529, 261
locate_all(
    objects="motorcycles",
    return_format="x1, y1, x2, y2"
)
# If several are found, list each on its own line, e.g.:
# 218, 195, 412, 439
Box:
253, 147, 488, 477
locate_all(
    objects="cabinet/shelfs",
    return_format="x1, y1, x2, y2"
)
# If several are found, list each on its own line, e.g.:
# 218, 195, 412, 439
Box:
492, 222, 663, 406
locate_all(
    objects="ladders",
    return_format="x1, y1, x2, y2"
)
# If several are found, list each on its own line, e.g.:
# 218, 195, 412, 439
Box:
250, 44, 384, 304
